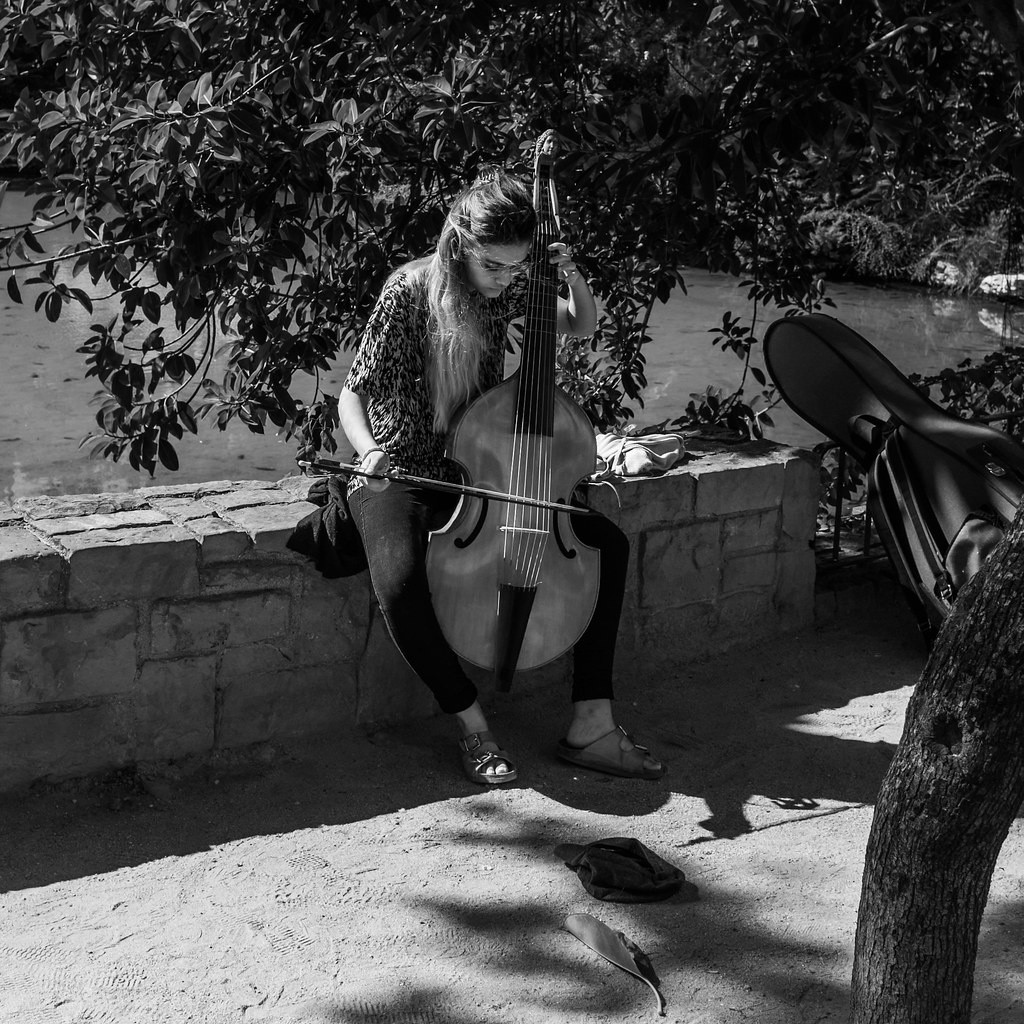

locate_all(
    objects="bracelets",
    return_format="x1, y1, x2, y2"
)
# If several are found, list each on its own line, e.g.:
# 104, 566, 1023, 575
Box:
359, 448, 387, 469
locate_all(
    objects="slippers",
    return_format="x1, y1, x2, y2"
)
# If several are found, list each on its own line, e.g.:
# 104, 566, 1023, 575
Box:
458, 731, 517, 784
556, 724, 667, 780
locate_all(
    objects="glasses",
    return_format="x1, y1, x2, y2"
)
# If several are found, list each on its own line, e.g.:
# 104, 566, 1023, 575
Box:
469, 252, 531, 277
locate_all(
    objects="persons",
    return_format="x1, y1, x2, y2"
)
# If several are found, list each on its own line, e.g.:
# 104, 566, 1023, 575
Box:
336, 160, 663, 785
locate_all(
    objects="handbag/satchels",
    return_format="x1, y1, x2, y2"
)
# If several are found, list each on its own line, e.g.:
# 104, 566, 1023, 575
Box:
579, 432, 685, 481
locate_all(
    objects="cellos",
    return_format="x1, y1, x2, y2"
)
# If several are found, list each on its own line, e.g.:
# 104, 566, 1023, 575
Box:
421, 122, 604, 698
